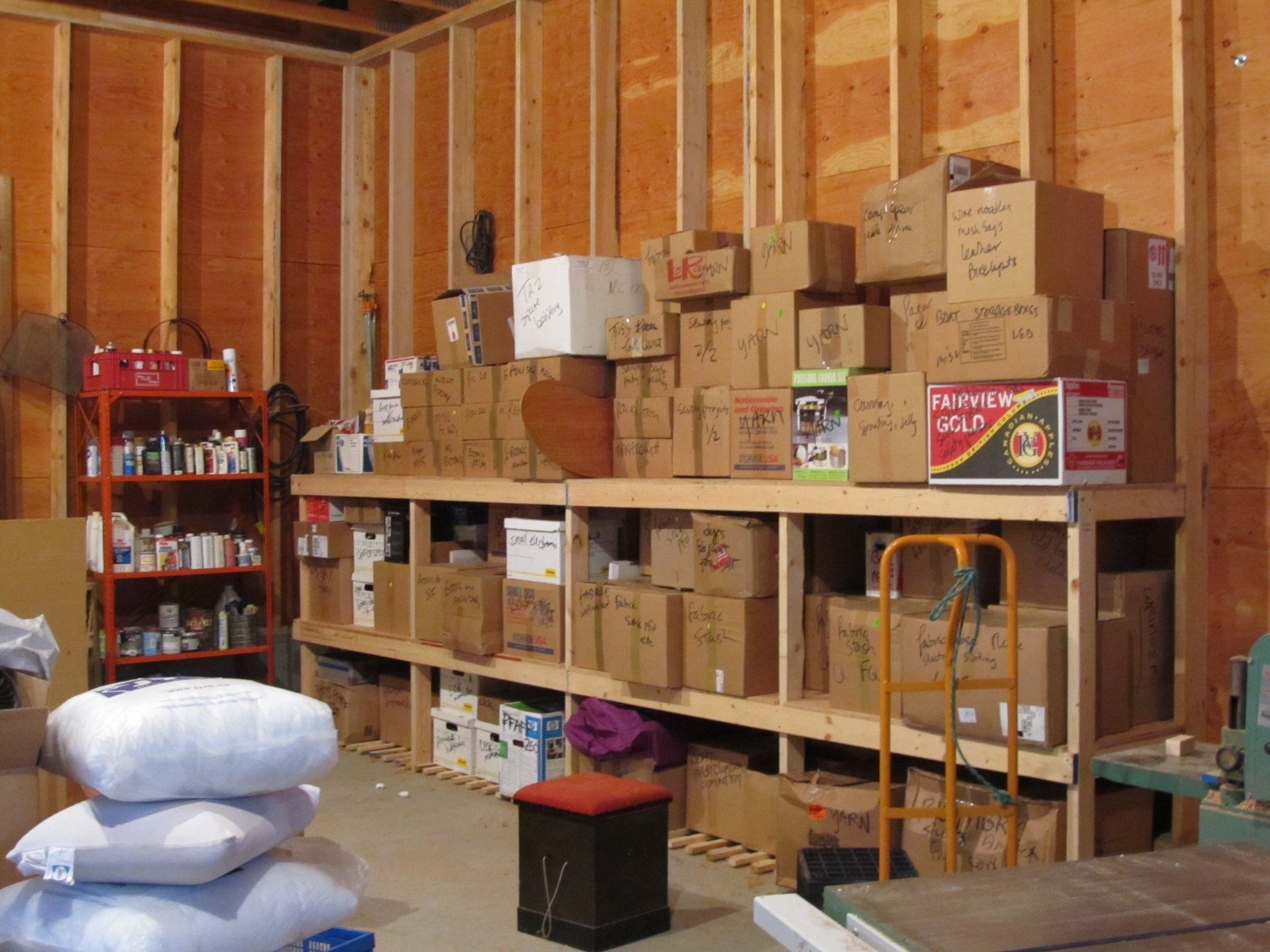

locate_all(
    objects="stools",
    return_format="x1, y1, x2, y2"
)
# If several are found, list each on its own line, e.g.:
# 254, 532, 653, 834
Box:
513, 771, 674, 952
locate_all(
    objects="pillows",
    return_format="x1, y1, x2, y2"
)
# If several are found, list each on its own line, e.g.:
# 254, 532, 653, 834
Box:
0, 836, 371, 952
5, 784, 321, 886
36, 676, 340, 804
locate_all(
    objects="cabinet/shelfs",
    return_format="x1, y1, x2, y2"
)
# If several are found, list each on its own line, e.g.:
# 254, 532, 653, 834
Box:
70, 387, 276, 687
290, 466, 1182, 888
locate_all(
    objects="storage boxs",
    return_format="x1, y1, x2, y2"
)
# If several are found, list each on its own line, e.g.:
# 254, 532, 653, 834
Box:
187, 357, 226, 392
294, 152, 1181, 876
0, 666, 49, 890
82, 349, 187, 391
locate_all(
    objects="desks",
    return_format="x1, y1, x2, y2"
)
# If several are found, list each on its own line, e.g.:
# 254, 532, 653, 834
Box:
753, 840, 1270, 951
